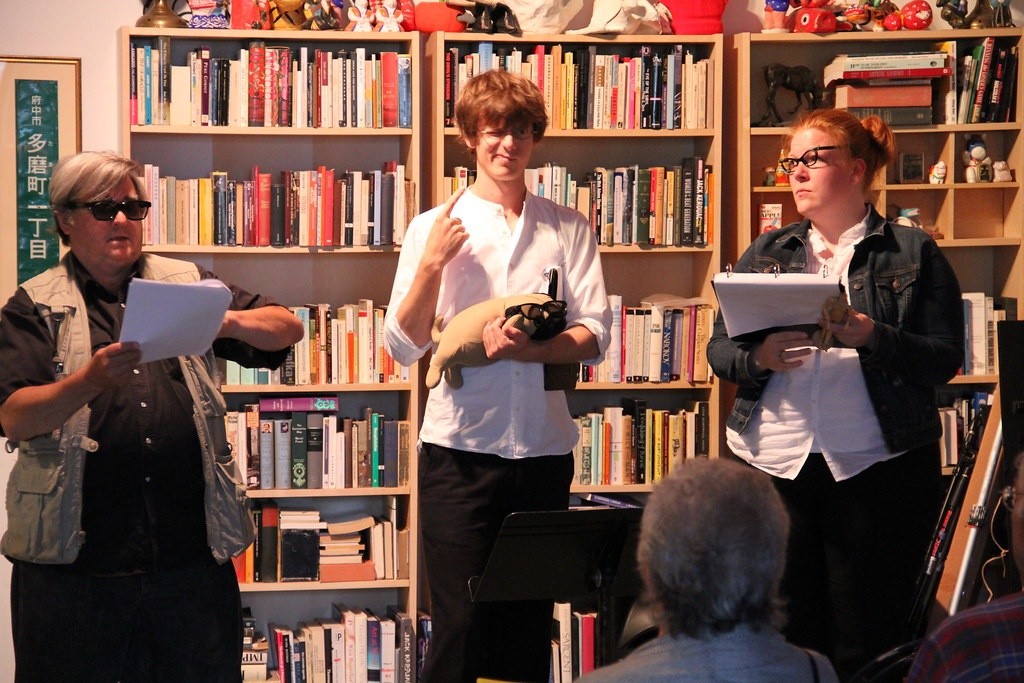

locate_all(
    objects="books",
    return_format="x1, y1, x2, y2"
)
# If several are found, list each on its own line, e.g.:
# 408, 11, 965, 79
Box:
120, 29, 1019, 682
712, 262, 848, 342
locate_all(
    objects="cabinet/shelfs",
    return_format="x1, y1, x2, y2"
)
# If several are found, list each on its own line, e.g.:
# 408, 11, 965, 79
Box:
733, 32, 1024, 475
126, 26, 422, 682
427, 29, 725, 496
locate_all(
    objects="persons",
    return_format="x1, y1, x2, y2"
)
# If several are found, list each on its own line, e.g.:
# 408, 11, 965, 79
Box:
345, 0, 405, 32
262, 424, 271, 433
569, 457, 838, 683
707, 108, 964, 683
0, 151, 304, 683
910, 450, 1024, 682
382, 69, 612, 683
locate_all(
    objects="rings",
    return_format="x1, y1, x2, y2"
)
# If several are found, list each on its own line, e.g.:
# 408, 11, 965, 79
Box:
777, 350, 785, 362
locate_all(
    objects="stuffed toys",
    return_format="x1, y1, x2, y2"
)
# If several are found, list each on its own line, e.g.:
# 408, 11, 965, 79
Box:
424, 293, 566, 389
760, 0, 934, 34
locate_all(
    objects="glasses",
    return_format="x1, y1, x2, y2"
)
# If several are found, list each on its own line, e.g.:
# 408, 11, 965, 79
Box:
65, 199, 152, 221
779, 146, 840, 173
998, 485, 1024, 511
478, 125, 538, 140
512, 301, 567, 320
